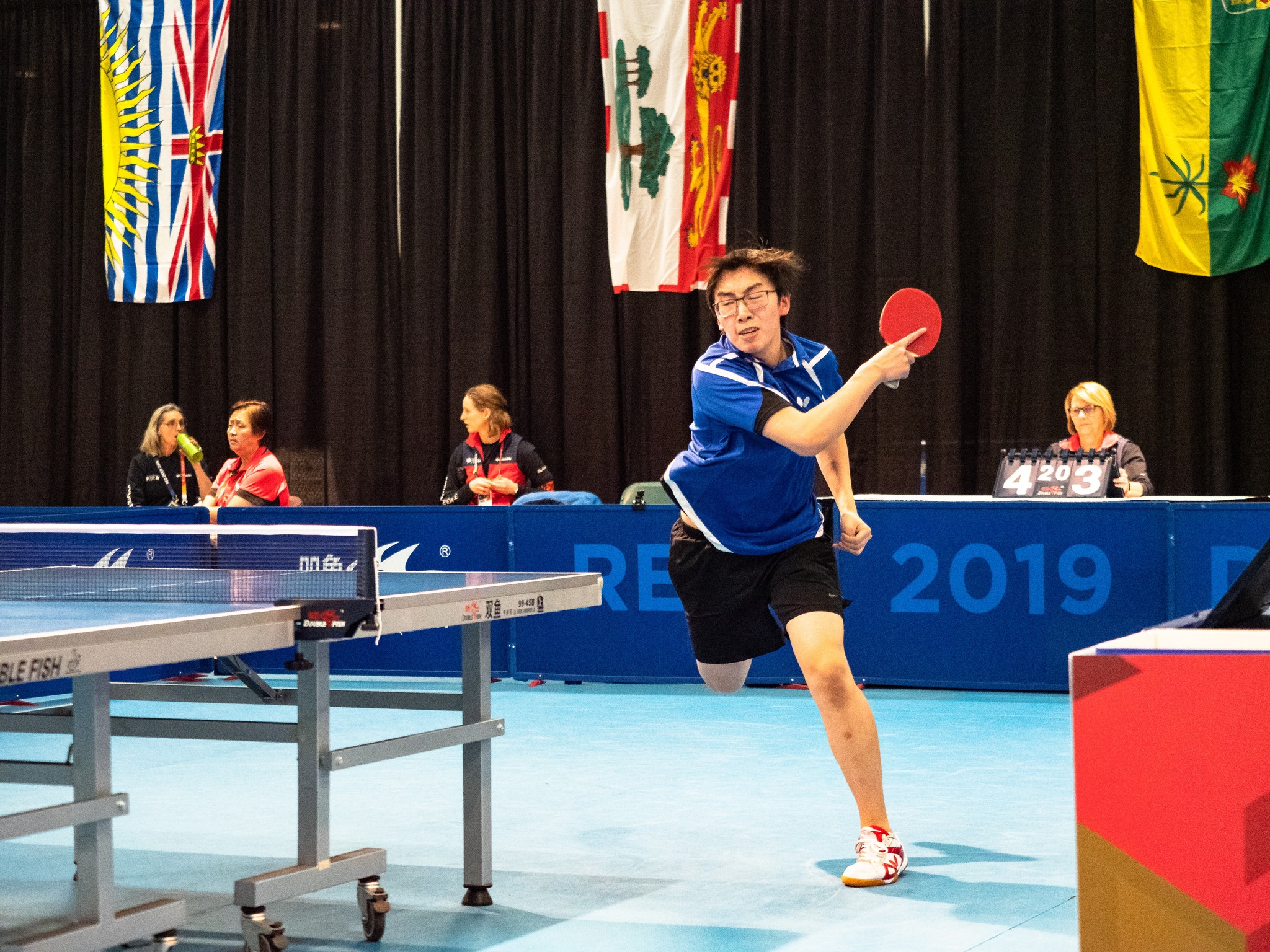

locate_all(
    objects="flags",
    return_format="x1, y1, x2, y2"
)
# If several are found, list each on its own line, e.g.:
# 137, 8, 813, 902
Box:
597, 0, 743, 295
1131, 0, 1270, 279
95, 0, 226, 304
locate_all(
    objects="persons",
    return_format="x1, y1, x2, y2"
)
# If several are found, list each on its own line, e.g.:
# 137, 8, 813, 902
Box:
660, 249, 909, 886
1039, 380, 1156, 501
439, 384, 554, 507
194, 400, 291, 507
125, 402, 212, 508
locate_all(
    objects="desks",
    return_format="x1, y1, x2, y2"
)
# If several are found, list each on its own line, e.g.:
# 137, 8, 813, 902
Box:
0, 558, 609, 950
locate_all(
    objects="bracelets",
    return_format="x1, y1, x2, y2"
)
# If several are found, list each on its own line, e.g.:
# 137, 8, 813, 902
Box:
1128, 481, 1130, 491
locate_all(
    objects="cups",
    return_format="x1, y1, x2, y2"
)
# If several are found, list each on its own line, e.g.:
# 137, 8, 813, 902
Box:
176, 434, 203, 463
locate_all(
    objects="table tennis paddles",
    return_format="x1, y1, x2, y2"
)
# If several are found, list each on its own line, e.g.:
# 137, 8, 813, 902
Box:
878, 288, 942, 388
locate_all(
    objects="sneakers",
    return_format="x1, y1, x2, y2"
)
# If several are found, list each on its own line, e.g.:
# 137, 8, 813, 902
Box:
840, 826, 908, 888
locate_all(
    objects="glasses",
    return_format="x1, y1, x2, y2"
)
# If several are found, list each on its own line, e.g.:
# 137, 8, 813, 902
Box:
159, 421, 188, 427
1068, 404, 1101, 416
711, 290, 778, 317
